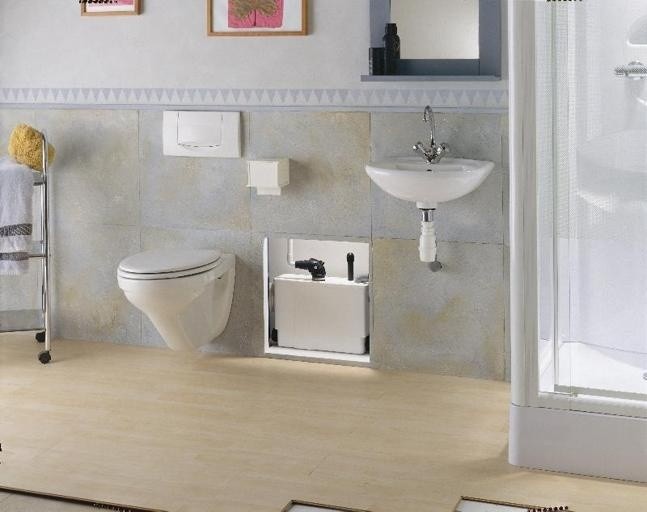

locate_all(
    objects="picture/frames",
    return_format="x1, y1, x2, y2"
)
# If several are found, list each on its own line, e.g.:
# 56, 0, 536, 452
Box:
80, 0, 140, 16
207, 0, 308, 36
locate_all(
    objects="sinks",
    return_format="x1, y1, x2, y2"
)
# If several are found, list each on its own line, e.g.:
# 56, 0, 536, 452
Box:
364, 158, 495, 201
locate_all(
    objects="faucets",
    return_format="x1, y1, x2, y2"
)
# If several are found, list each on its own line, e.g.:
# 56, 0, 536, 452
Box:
422, 104, 437, 155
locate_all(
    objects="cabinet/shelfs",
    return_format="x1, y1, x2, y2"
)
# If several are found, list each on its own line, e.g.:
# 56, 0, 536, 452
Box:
0, 128, 52, 364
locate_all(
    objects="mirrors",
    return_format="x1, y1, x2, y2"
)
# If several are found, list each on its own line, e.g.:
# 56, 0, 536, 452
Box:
361, 0, 502, 82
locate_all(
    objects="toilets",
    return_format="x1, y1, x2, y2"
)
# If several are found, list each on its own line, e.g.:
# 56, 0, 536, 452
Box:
117, 249, 235, 350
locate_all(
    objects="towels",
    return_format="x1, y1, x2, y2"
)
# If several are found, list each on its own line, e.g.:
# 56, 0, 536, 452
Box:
0, 155, 34, 277
